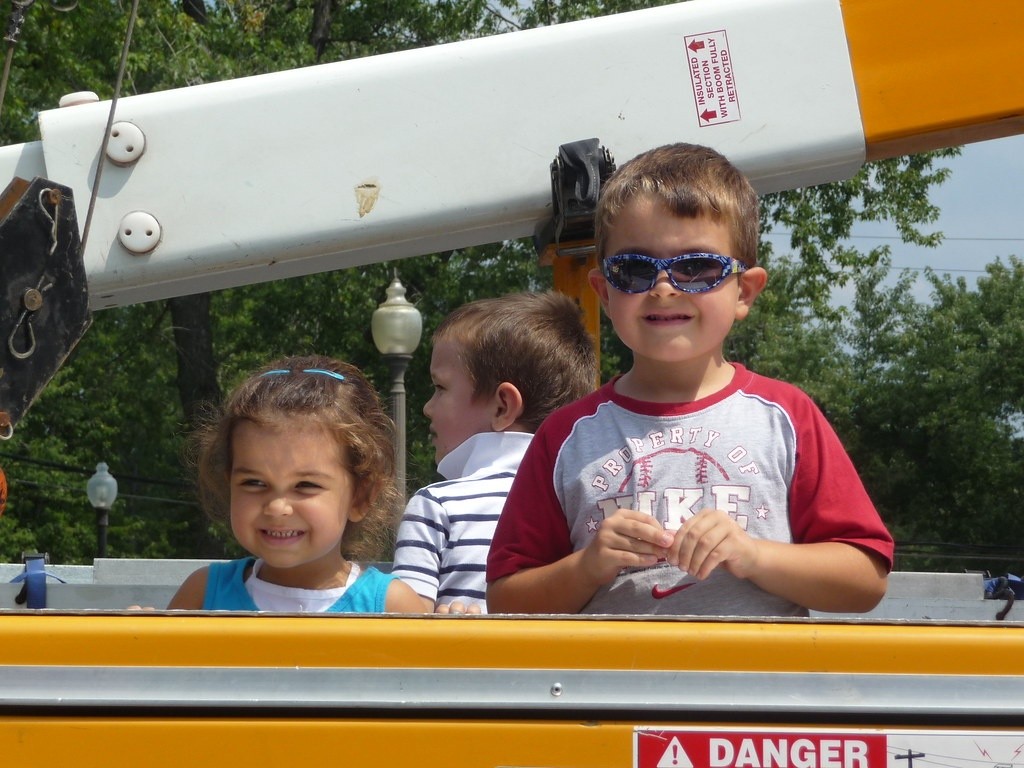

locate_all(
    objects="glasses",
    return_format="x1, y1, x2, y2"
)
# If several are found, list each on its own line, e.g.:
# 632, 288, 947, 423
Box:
599, 252, 747, 294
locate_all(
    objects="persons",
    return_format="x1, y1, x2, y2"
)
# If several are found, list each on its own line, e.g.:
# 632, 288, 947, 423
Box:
482, 140, 898, 623
162, 354, 483, 615
388, 285, 604, 615
992, 576, 1012, 602
984, 569, 1016, 601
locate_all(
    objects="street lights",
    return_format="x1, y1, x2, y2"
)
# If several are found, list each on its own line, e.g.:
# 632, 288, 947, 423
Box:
87, 463, 119, 559
368, 266, 425, 517
